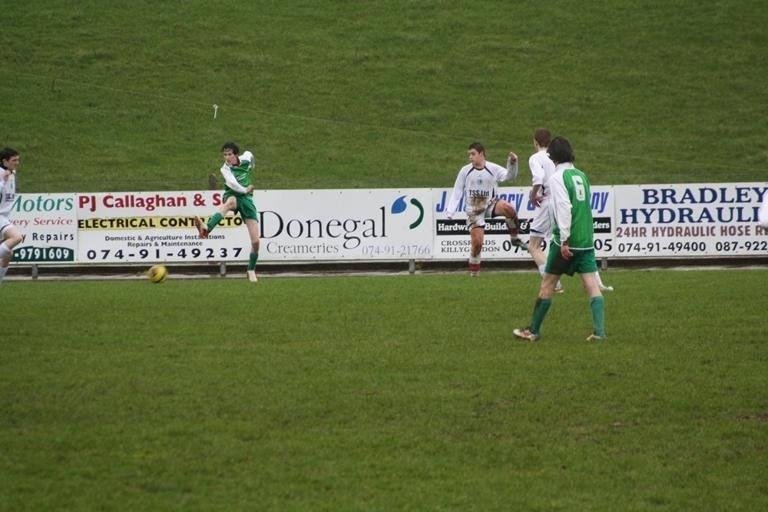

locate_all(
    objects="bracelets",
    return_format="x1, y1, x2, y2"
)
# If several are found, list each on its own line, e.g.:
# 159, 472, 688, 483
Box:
560, 241, 568, 246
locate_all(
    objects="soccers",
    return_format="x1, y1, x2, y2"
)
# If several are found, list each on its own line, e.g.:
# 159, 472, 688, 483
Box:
147, 263, 168, 282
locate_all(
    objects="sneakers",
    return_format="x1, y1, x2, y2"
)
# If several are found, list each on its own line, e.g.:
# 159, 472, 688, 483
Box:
513, 328, 538, 341
599, 285, 613, 292
194, 215, 208, 238
555, 283, 563, 293
511, 238, 529, 249
246, 270, 257, 282
587, 333, 602, 341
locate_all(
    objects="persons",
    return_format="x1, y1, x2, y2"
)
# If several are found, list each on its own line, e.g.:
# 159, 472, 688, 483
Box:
512, 135, 605, 342
445, 142, 529, 277
526, 128, 614, 294
0, 147, 23, 280
194, 142, 261, 283
